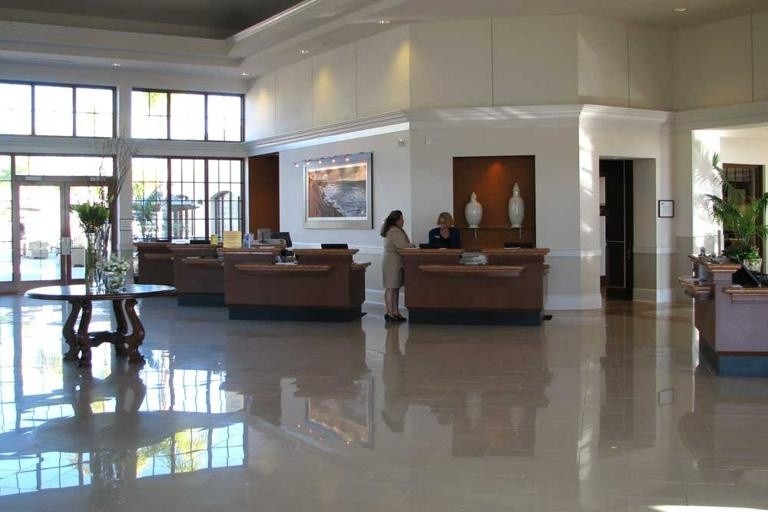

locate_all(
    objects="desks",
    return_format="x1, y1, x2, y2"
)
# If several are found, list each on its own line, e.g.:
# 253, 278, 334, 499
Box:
24, 283, 177, 368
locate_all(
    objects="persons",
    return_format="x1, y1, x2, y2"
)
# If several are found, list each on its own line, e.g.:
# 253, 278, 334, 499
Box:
379, 210, 416, 322
381, 319, 409, 435
428, 212, 462, 250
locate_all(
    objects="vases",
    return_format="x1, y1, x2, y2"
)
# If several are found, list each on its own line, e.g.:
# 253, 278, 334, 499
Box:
464, 182, 525, 229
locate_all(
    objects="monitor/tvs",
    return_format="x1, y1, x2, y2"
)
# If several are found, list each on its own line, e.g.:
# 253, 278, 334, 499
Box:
321, 243, 348, 249
503, 243, 533, 249
740, 263, 762, 287
270, 232, 292, 248
419, 243, 450, 249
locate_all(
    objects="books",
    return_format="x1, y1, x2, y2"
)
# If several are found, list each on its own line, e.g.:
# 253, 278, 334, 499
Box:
504, 246, 521, 250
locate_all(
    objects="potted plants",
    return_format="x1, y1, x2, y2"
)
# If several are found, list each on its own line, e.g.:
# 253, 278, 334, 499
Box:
68, 128, 133, 290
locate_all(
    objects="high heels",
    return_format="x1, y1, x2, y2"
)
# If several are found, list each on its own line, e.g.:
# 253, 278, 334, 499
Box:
384, 313, 407, 321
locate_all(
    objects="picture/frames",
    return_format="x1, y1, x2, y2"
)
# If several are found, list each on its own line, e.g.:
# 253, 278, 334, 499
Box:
658, 200, 674, 218
